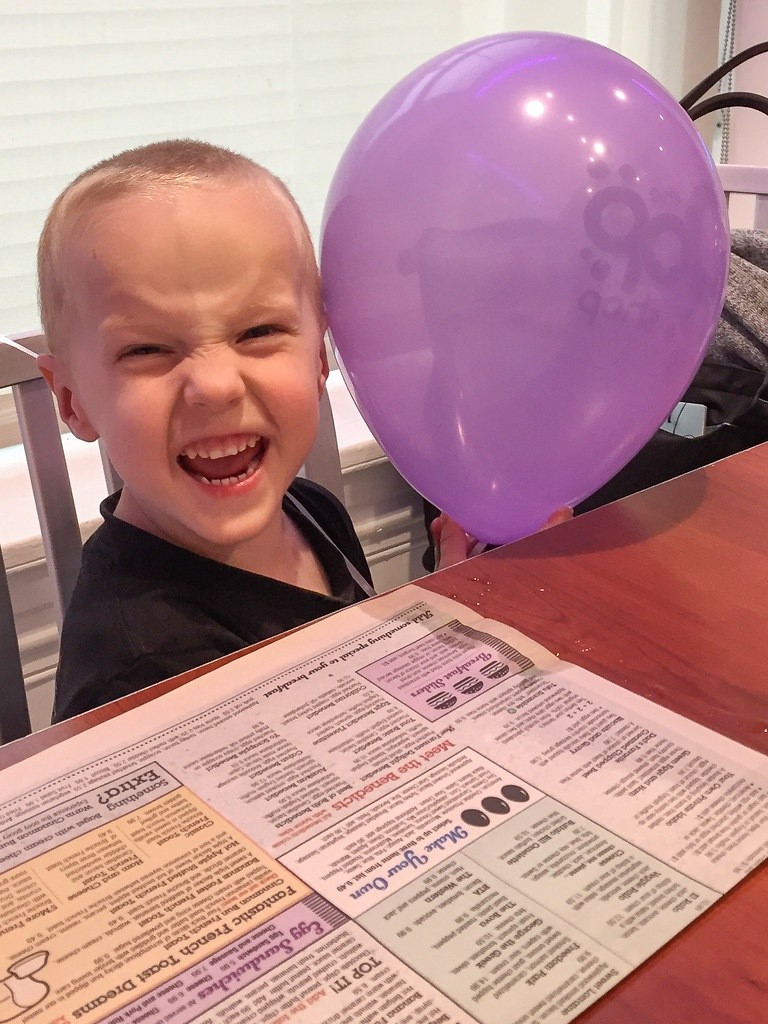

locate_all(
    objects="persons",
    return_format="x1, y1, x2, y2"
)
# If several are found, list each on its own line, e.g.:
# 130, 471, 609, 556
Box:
37, 138, 571, 726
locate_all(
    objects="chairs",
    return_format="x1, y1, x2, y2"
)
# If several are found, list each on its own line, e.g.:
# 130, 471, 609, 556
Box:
0, 333, 344, 746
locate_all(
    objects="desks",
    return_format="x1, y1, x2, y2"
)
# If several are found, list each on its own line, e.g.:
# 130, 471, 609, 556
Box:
0, 441, 768, 1024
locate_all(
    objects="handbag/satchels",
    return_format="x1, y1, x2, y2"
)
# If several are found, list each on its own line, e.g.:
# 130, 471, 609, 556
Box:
421, 42, 768, 570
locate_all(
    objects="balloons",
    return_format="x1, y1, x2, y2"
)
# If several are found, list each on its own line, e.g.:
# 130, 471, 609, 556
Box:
321, 34, 729, 546
